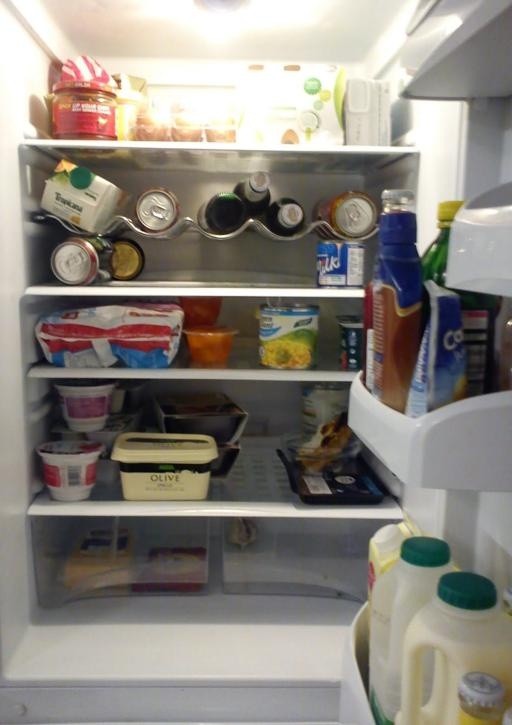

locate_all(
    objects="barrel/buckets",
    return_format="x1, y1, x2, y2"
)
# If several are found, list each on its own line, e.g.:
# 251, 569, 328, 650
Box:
393, 571, 511, 725
366, 535, 454, 725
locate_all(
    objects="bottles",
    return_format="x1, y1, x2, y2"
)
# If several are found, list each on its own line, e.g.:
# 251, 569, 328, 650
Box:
370, 188, 502, 411
197, 170, 304, 238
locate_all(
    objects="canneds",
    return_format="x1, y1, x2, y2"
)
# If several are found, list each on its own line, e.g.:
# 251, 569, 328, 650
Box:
135, 184, 181, 234
47, 232, 114, 287
301, 382, 350, 443
312, 191, 379, 241
259, 297, 320, 370
109, 238, 145, 281
